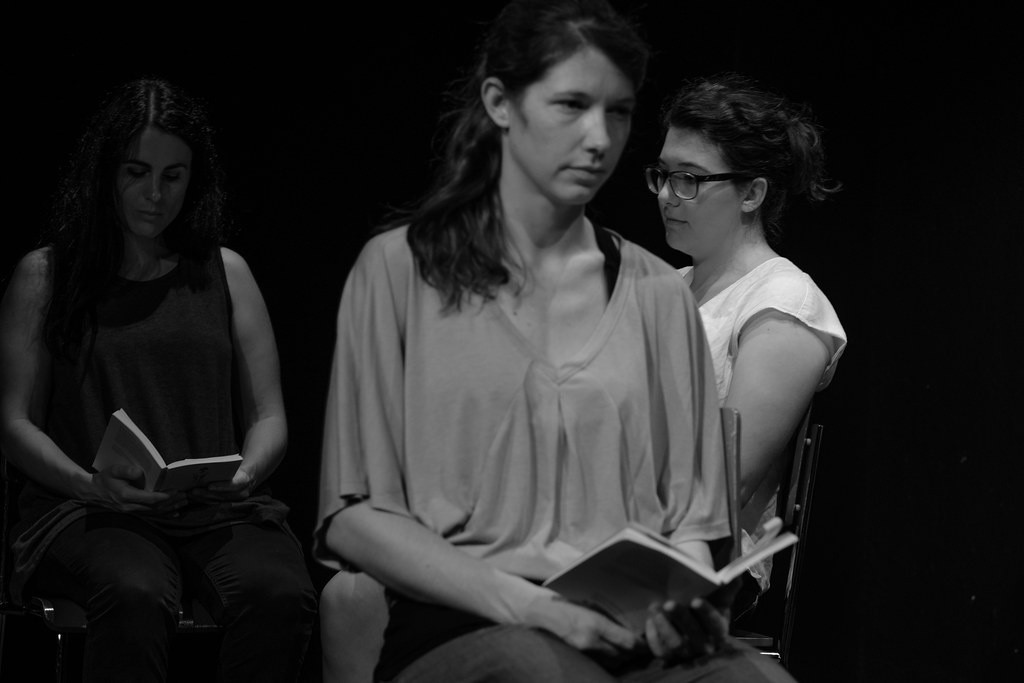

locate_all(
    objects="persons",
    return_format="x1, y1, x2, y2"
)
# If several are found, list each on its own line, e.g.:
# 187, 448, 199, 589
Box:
643, 79, 848, 631
316, 1, 801, 681
1, 80, 317, 683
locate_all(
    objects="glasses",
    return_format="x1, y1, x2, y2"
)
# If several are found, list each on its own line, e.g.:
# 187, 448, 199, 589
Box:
645, 165, 757, 200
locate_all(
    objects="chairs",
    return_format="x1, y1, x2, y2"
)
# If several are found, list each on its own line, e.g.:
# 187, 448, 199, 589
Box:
22, 589, 223, 652
727, 419, 824, 666
